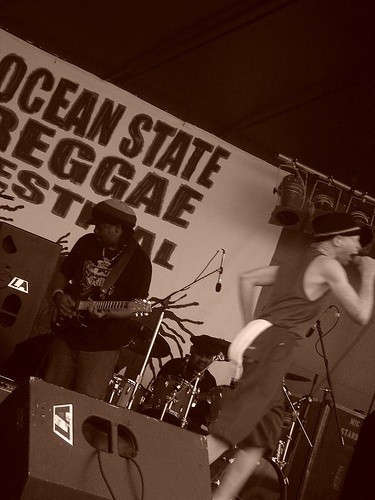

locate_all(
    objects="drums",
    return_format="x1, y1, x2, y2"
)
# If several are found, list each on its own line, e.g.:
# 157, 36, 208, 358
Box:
141, 374, 201, 424
204, 385, 231, 424
211, 455, 289, 500
104, 372, 151, 413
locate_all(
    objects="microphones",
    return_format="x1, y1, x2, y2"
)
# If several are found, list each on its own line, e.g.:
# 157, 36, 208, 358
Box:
353, 256, 362, 264
306, 305, 332, 337
215, 250, 225, 292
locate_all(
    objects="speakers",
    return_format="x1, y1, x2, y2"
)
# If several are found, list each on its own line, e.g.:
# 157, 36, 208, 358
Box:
0, 376, 212, 500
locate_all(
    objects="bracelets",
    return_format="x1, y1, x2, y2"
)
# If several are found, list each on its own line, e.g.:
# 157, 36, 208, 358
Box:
52, 290, 63, 302
104, 310, 111, 321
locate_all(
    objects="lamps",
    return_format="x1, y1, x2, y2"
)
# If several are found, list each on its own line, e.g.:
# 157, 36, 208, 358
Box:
269, 175, 375, 255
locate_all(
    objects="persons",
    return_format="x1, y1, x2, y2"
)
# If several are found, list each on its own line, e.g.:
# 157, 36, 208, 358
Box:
151, 344, 216, 437
206, 212, 375, 500
51, 199, 152, 400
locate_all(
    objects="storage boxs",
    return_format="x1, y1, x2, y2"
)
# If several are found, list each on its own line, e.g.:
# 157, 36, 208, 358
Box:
283, 391, 366, 500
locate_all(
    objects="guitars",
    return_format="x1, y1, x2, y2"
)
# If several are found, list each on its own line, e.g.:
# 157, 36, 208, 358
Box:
50, 285, 152, 331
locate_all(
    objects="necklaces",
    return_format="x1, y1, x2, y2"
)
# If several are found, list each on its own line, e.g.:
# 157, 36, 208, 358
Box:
102, 247, 121, 262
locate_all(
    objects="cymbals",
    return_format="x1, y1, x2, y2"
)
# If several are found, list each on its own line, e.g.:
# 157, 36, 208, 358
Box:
127, 327, 171, 358
190, 336, 231, 362
